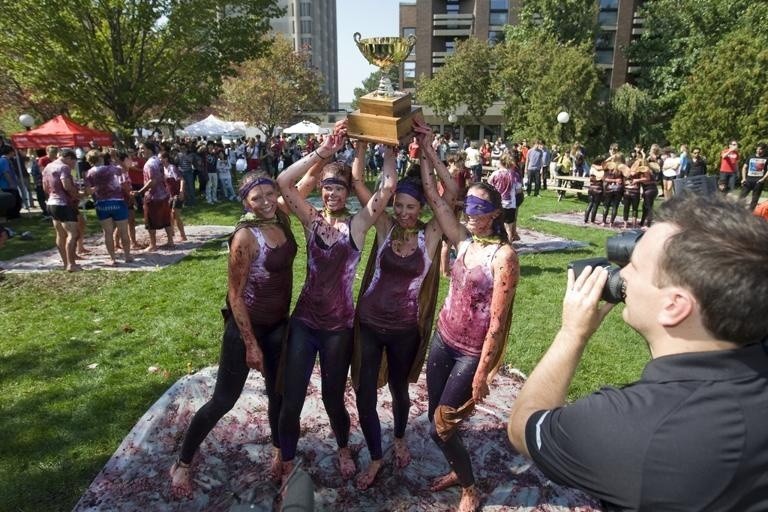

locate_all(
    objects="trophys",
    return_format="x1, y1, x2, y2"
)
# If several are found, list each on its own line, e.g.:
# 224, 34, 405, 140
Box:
342, 30, 429, 148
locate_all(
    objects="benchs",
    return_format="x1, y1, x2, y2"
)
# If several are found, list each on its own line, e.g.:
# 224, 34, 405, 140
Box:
546, 186, 590, 206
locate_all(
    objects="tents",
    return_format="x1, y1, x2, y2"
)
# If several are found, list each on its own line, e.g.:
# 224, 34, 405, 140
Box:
12, 114, 113, 223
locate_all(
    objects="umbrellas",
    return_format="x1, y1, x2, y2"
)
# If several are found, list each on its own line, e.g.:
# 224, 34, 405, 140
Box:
130, 115, 332, 137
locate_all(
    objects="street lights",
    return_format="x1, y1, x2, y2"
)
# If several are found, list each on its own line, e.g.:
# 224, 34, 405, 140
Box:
19, 113, 35, 132
447, 112, 458, 139
557, 110, 571, 145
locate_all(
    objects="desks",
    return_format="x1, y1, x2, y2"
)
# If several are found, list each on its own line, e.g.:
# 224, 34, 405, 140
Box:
555, 176, 590, 204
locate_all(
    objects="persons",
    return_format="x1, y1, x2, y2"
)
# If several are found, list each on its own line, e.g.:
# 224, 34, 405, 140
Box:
407, 111, 521, 511
1, 127, 768, 272
168, 121, 348, 497
352, 117, 458, 488
508, 188, 768, 511
274, 121, 398, 490
